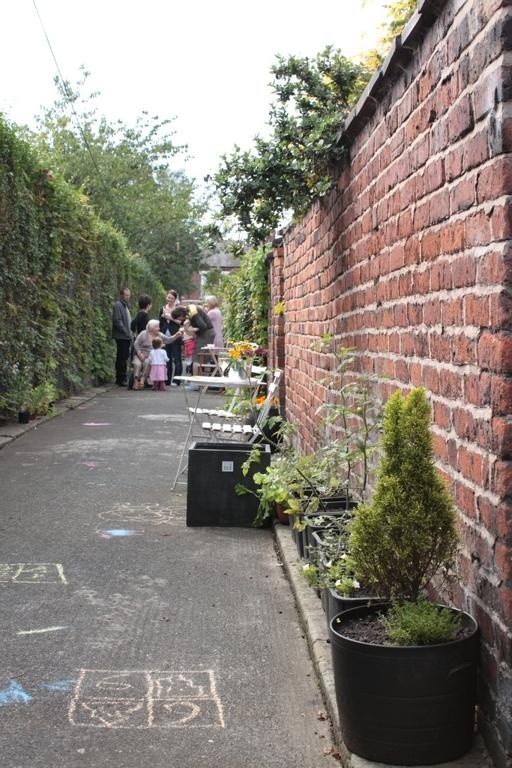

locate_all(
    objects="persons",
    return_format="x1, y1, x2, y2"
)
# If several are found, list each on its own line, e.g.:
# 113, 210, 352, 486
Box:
112, 287, 133, 386
130, 288, 223, 391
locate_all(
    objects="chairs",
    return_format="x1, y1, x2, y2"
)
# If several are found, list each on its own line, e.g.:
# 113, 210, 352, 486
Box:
172, 341, 282, 485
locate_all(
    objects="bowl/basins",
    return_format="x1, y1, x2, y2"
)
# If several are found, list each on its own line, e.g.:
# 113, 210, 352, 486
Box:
207, 343, 215, 348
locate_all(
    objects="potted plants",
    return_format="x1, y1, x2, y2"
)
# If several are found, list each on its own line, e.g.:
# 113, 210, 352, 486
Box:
326, 384, 478, 763
11, 388, 51, 423
228, 326, 405, 633
